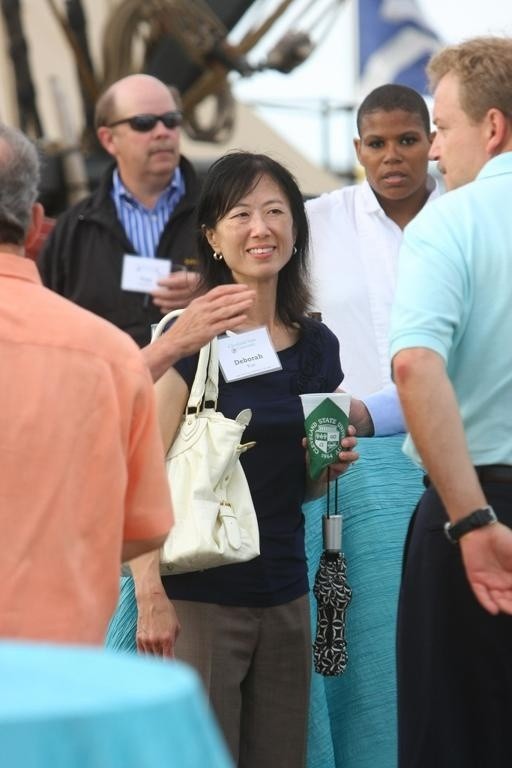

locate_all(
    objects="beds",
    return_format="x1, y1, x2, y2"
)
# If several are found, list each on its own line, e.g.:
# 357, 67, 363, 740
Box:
313, 466, 351, 677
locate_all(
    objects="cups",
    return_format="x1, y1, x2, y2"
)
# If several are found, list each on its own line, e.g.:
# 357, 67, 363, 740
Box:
104, 111, 184, 132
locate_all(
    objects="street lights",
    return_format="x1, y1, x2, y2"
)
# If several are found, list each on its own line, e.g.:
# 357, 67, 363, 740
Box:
116, 309, 261, 576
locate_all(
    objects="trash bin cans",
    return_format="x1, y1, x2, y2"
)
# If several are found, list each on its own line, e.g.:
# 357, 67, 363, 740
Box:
443, 506, 496, 543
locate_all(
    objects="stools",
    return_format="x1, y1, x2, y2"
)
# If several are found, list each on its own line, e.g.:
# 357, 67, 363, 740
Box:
480, 465, 512, 481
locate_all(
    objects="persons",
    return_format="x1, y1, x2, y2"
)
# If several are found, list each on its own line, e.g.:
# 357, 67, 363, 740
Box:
303, 87, 442, 401
0, 122, 175, 648
137, 282, 254, 385
390, 36, 512, 767
35, 75, 201, 347
127, 155, 359, 767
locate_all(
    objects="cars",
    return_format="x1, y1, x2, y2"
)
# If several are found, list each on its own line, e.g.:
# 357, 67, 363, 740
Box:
298, 392, 354, 482
